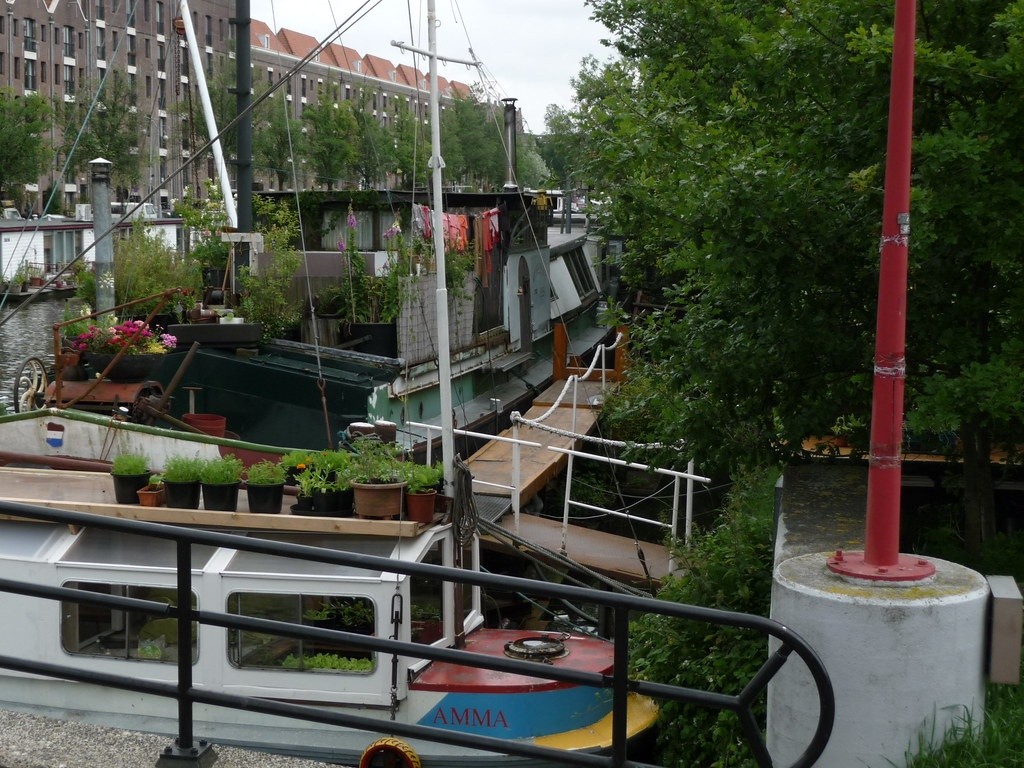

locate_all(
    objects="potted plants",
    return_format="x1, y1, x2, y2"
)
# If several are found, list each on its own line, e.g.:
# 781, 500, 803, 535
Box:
110, 450, 151, 504
200, 453, 242, 511
349, 437, 408, 520
0, 268, 85, 294
245, 457, 285, 513
313, 447, 354, 515
305, 599, 427, 641
136, 473, 167, 507
338, 203, 399, 358
406, 462, 437, 524
161, 453, 201, 508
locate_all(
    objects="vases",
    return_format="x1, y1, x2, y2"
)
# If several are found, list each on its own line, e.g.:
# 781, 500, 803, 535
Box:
296, 493, 312, 512
82, 349, 168, 383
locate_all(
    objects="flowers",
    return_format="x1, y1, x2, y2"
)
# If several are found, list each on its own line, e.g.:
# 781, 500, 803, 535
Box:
69, 320, 178, 360
295, 452, 329, 472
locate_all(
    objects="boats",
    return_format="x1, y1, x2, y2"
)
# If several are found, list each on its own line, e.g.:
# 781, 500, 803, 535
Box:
1, 406, 437, 480
0, 519, 658, 759
40, 188, 621, 470
